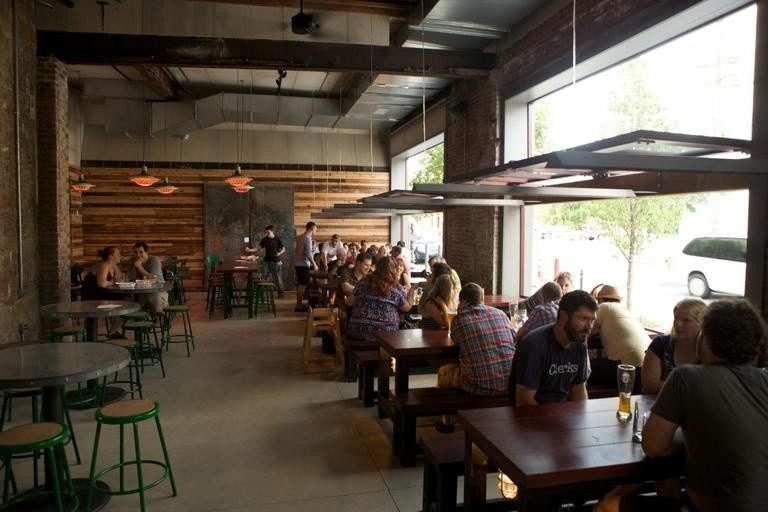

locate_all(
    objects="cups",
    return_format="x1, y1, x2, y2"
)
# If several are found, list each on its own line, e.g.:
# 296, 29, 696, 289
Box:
615, 364, 635, 418
632, 400, 648, 440
509, 300, 527, 325
447, 303, 455, 338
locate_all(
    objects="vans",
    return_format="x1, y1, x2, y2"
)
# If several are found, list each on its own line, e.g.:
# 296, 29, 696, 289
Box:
673, 234, 748, 302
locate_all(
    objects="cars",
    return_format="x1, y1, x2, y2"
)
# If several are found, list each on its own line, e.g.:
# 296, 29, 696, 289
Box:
408, 236, 441, 266
535, 221, 613, 243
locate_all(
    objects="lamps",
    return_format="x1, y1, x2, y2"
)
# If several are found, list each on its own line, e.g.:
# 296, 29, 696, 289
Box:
69, 74, 255, 195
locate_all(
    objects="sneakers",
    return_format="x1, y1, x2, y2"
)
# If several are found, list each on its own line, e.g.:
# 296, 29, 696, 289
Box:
434, 419, 455, 433
293, 302, 309, 312
107, 331, 128, 340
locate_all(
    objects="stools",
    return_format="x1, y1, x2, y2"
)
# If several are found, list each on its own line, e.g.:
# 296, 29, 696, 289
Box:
204, 256, 275, 319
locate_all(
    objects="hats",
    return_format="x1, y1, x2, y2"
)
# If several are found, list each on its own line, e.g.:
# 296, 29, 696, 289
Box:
595, 283, 623, 301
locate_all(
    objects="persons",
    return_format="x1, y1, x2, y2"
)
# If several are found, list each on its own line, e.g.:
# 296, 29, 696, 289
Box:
641, 299, 707, 393
127, 242, 169, 333
594, 298, 768, 512
435, 283, 518, 433
245, 225, 285, 298
81, 247, 127, 340
294, 222, 318, 312
509, 290, 593, 512
514, 282, 563, 340
311, 234, 461, 379
588, 285, 652, 389
519, 272, 574, 316
